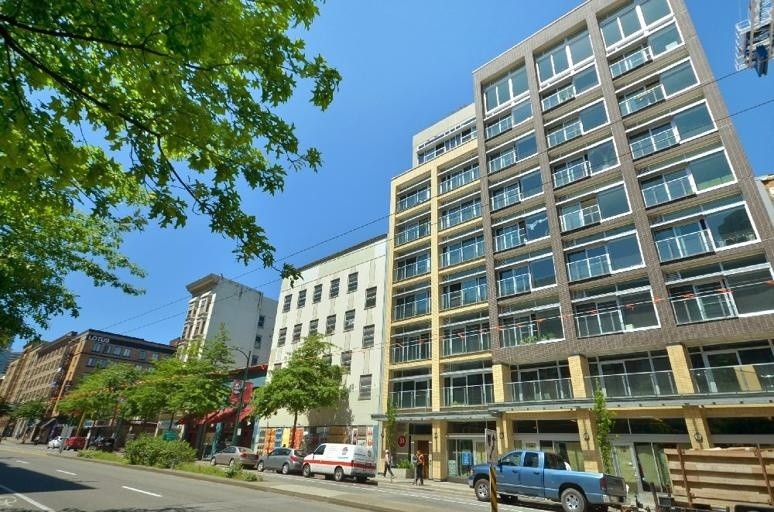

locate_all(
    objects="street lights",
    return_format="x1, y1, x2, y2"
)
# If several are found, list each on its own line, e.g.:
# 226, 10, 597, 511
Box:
228, 346, 251, 445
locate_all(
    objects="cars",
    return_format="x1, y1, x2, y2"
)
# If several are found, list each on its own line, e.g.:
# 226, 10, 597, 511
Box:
209, 446, 258, 469
46, 435, 114, 452
255, 447, 305, 475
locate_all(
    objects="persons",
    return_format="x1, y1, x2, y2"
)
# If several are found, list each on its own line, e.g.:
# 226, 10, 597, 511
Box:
416, 450, 424, 484
562, 458, 571, 470
382, 449, 394, 478
412, 449, 420, 485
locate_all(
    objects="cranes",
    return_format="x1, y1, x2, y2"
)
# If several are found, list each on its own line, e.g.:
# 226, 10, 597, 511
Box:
735, 2, 772, 76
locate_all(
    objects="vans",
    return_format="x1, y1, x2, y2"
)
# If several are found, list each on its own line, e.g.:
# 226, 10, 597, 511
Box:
300, 443, 376, 482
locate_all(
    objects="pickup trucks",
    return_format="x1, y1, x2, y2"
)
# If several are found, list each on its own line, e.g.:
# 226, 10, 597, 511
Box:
468, 449, 627, 512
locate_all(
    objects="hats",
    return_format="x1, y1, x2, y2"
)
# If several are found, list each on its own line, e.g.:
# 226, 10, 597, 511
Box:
385, 450, 390, 453
